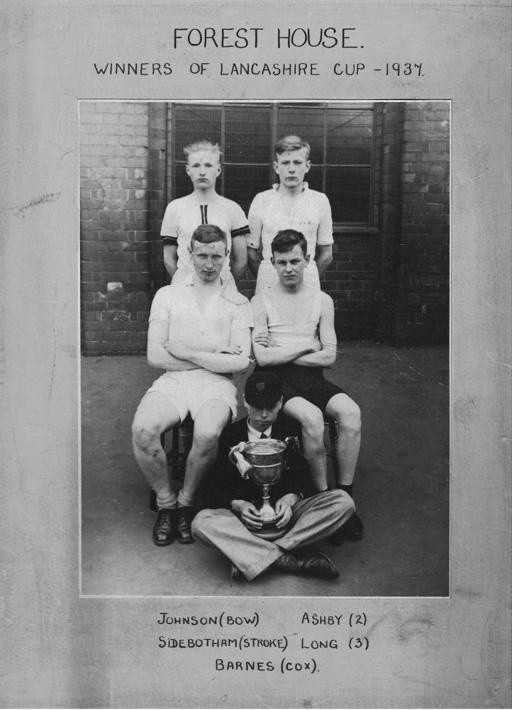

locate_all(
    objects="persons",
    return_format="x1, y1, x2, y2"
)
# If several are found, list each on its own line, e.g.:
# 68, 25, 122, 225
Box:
244, 135, 335, 297
189, 371, 357, 579
160, 141, 250, 285
131, 224, 255, 546
251, 229, 364, 546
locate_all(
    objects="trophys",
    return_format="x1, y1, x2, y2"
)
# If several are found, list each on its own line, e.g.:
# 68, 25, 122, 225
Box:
228, 436, 299, 542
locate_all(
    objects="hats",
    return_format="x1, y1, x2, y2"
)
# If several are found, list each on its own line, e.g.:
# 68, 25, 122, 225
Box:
245, 373, 283, 409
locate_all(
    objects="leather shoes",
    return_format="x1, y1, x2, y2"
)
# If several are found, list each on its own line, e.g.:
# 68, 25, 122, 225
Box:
153, 510, 175, 545
176, 508, 194, 543
280, 550, 339, 579
343, 511, 364, 539
